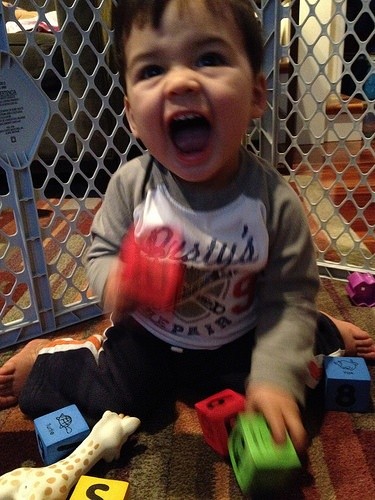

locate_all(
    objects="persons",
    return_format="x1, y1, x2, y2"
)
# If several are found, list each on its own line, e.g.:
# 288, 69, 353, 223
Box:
1, 0, 375, 449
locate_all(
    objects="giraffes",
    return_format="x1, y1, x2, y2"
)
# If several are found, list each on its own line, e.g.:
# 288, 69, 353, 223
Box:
0, 410, 141, 500
